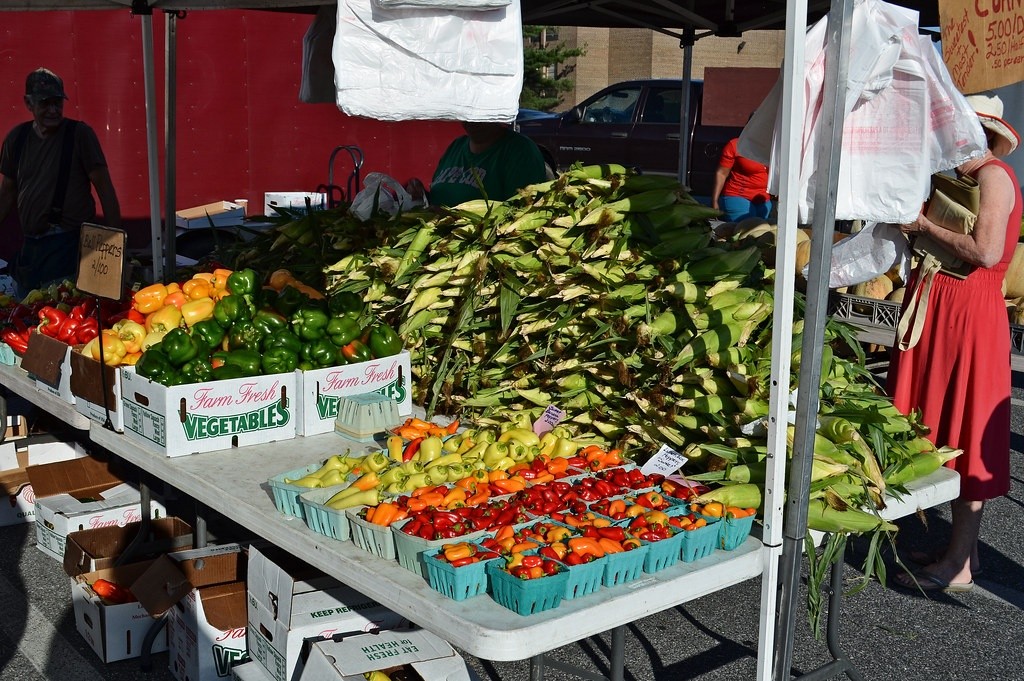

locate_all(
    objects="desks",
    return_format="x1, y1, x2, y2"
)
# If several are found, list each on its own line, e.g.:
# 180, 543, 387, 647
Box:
0, 340, 962, 661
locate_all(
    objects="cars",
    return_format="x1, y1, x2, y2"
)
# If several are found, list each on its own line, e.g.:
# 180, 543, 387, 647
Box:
515, 74, 745, 197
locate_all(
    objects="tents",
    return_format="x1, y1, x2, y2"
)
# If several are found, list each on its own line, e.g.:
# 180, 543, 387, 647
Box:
0, 0, 941, 681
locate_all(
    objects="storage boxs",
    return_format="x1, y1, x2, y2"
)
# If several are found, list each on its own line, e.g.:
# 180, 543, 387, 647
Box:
0, 418, 472, 681
29, 327, 124, 434
293, 348, 413, 438
120, 359, 298, 458
829, 290, 1024, 356
263, 191, 327, 218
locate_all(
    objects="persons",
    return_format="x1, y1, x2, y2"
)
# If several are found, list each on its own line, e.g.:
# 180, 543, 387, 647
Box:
887, 95, 1023, 592
400, 122, 547, 211
711, 107, 772, 223
0, 66, 123, 298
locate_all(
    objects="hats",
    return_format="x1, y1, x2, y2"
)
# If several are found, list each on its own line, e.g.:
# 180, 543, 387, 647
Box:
24, 67, 72, 103
963, 89, 1021, 157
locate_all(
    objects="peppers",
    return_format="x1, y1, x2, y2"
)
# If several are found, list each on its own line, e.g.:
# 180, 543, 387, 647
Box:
86, 579, 138, 605
283, 414, 756, 581
0, 268, 403, 386
364, 671, 392, 681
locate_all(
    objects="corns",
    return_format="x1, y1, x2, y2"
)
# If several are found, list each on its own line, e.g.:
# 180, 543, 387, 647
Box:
213, 163, 964, 532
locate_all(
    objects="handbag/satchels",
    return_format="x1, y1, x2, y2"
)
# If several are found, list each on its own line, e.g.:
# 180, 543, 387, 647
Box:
912, 172, 981, 280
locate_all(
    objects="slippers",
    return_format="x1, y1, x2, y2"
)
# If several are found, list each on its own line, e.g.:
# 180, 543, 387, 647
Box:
891, 547, 986, 592
